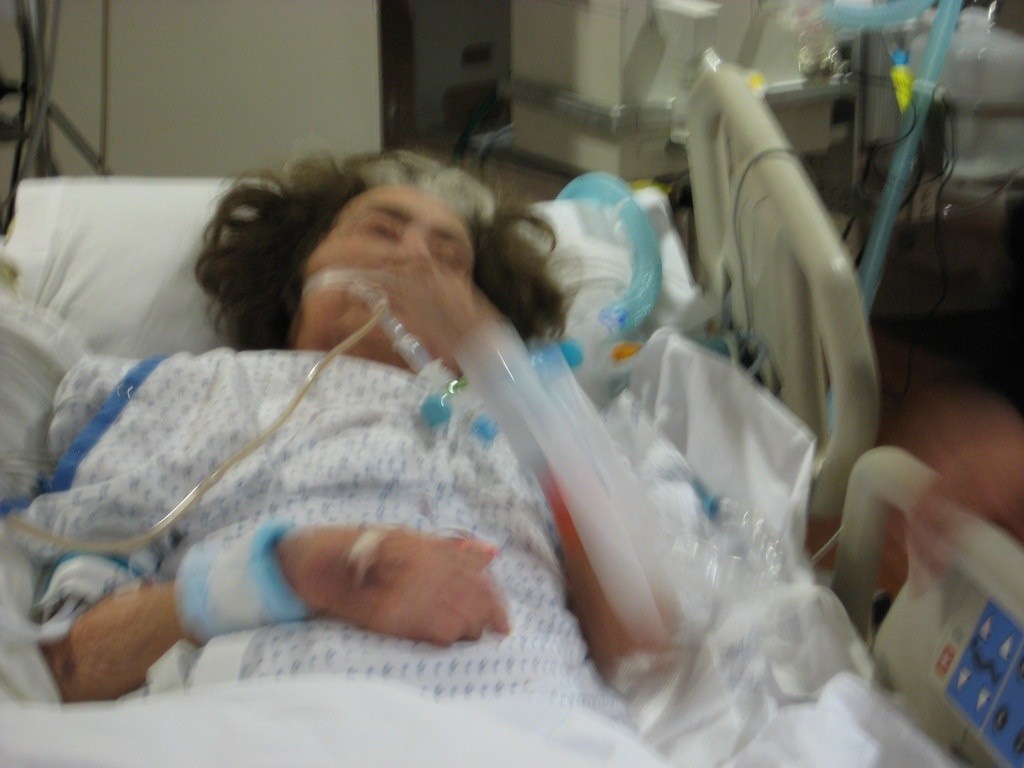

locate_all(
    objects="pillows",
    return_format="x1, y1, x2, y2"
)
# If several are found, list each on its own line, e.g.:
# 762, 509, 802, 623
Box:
43, 180, 622, 365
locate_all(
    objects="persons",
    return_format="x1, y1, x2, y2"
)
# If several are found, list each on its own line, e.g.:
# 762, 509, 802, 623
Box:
29, 149, 683, 768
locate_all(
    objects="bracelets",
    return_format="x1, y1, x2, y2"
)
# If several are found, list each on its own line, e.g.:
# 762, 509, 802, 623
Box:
174, 519, 309, 646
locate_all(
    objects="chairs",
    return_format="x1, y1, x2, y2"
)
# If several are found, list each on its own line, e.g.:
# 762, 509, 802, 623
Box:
0, 49, 1024, 768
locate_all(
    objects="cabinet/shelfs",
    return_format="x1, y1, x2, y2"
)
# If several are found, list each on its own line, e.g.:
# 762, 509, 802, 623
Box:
465, 1, 844, 278
